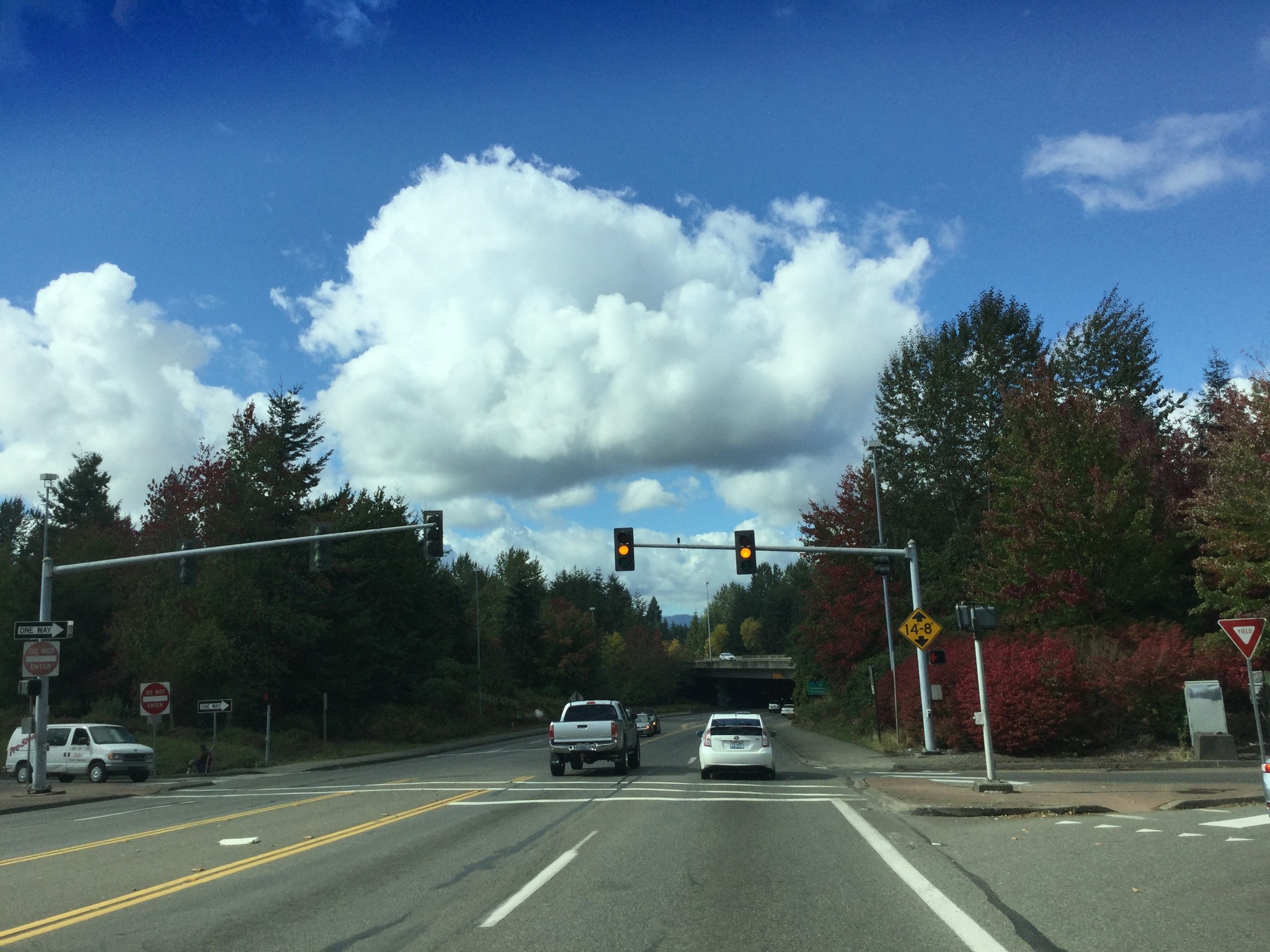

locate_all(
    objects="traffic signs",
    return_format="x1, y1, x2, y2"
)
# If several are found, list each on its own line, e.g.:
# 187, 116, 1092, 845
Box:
197, 699, 232, 713
13, 621, 74, 640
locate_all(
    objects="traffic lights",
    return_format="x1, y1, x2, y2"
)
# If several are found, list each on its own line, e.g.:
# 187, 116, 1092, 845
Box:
734, 529, 758, 575
613, 527, 635, 571
929, 649, 947, 664
422, 510, 444, 557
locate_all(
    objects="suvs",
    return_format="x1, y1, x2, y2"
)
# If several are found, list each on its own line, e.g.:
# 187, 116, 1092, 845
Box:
769, 700, 782, 713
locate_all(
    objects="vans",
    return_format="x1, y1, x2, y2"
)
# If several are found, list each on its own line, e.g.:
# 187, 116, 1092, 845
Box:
6, 725, 154, 783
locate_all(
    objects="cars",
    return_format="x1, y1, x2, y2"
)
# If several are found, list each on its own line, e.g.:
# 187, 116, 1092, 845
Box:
781, 704, 794, 716
625, 710, 661, 737
696, 713, 777, 779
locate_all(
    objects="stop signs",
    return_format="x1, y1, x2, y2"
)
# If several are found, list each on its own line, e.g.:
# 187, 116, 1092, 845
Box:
22, 641, 60, 677
140, 682, 170, 716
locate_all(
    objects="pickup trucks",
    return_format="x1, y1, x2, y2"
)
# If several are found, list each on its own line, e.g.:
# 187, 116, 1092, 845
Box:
548, 700, 641, 777
